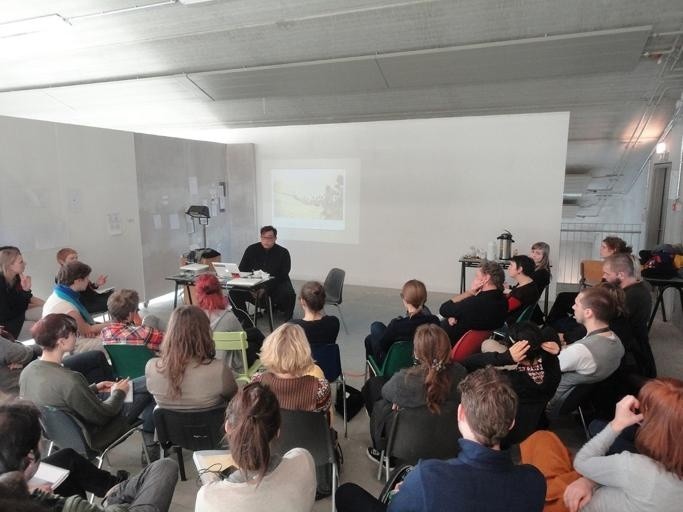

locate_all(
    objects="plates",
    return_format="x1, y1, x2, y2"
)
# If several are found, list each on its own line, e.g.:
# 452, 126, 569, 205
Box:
252, 275, 269, 281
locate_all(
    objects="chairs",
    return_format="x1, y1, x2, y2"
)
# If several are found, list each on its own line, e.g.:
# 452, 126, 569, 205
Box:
578, 260, 606, 290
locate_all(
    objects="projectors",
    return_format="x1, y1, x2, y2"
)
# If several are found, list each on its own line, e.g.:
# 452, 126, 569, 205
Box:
179, 263, 209, 279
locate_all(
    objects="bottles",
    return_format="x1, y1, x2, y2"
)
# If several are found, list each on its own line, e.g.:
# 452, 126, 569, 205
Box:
496, 233, 515, 260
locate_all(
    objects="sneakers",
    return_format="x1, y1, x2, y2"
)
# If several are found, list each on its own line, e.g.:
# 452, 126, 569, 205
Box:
100, 470, 128, 498
141, 440, 161, 465
366, 446, 396, 472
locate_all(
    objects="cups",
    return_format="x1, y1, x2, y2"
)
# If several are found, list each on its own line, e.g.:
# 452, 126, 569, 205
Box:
255, 271, 268, 278
468, 245, 490, 262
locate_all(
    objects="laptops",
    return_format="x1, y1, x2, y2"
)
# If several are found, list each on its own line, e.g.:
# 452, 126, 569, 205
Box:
212, 262, 253, 278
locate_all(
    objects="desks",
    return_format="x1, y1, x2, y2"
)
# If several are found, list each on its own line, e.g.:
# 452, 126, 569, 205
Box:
456, 256, 550, 313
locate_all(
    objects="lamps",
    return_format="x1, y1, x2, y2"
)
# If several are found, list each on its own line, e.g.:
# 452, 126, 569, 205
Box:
186, 204, 211, 253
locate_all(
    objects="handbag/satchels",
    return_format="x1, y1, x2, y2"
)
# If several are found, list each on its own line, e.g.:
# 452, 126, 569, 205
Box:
335, 383, 365, 422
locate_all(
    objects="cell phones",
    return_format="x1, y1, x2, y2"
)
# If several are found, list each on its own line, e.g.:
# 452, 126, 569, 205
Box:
219, 465, 239, 478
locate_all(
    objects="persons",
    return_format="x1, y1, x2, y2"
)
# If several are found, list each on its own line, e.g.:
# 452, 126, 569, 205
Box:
0, 244, 341, 512
227, 226, 294, 329
333, 237, 682, 512
